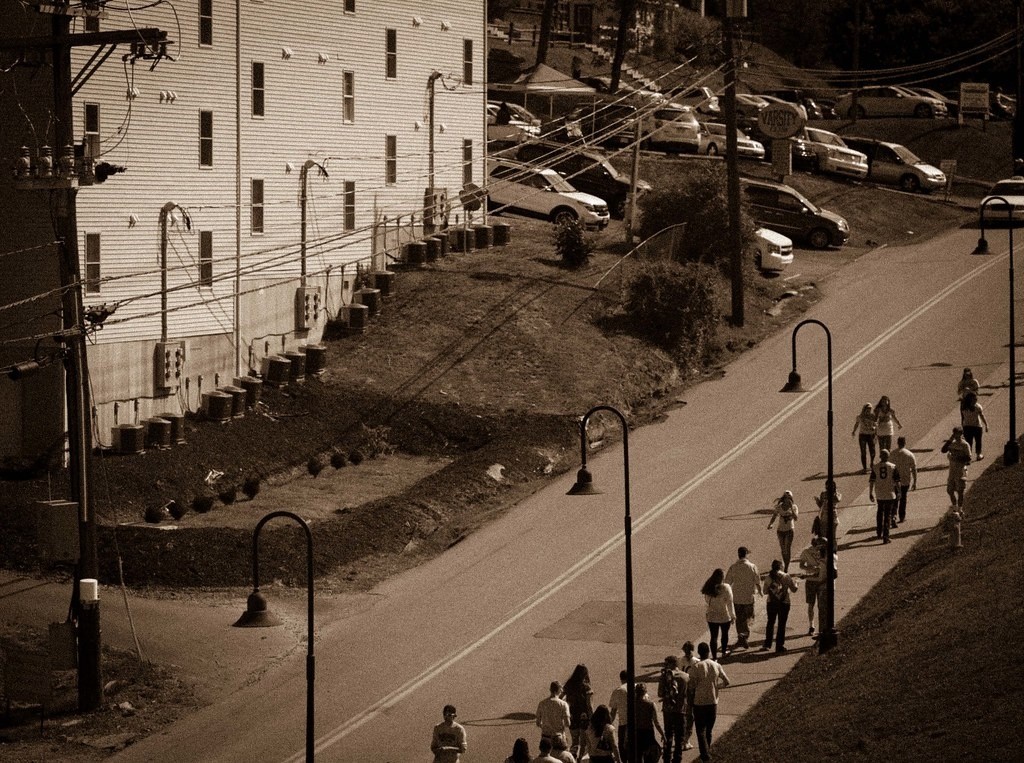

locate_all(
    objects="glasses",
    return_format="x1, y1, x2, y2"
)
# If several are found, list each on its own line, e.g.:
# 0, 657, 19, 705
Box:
444, 713, 455, 716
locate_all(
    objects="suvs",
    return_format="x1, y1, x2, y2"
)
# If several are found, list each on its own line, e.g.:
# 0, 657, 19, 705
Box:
488, 157, 610, 229
521, 142, 653, 218
627, 185, 796, 270
640, 107, 701, 152
660, 86, 720, 115
569, 102, 639, 145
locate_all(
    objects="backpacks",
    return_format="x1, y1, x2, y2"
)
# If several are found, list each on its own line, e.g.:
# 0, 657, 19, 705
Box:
770, 574, 783, 595
664, 678, 686, 712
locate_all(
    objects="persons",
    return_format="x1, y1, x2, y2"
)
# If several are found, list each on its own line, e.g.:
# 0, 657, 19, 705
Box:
701, 568, 736, 661
430, 705, 467, 763
874, 395, 902, 460
724, 546, 763, 649
815, 480, 842, 553
796, 539, 838, 635
955, 367, 980, 403
890, 437, 918, 528
941, 426, 972, 516
767, 490, 799, 573
851, 403, 877, 474
764, 559, 798, 652
496, 101, 512, 125
867, 449, 901, 544
504, 738, 533, 763
570, 56, 582, 79
960, 392, 988, 461
533, 642, 730, 763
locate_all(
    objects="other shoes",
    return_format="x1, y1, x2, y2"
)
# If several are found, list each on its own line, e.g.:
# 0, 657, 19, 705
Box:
739, 634, 748, 649
892, 518, 906, 528
763, 645, 770, 651
977, 454, 984, 460
722, 651, 730, 657
682, 743, 693, 750
776, 647, 786, 652
876, 534, 891, 543
953, 506, 965, 514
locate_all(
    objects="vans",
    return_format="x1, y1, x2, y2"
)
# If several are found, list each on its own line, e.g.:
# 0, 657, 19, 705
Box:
741, 178, 850, 248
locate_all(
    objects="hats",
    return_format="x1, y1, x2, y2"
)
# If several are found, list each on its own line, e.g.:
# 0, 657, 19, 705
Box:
964, 368, 971, 375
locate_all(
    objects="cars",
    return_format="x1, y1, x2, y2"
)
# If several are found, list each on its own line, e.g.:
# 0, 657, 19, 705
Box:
799, 127, 869, 180
841, 135, 947, 192
835, 86, 1014, 119
741, 121, 818, 168
582, 77, 667, 108
720, 93, 842, 119
698, 121, 766, 160
978, 177, 1024, 223
487, 102, 543, 142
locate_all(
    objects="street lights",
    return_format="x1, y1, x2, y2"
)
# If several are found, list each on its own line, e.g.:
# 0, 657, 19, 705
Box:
231, 512, 315, 763
567, 407, 639, 760
778, 319, 836, 650
971, 196, 1021, 466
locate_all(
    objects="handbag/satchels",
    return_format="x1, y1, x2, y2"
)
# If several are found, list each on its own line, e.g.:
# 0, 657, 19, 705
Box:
812, 516, 820, 535
598, 736, 612, 751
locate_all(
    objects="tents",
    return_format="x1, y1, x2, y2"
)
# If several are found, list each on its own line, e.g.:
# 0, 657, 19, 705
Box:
488, 63, 596, 150
